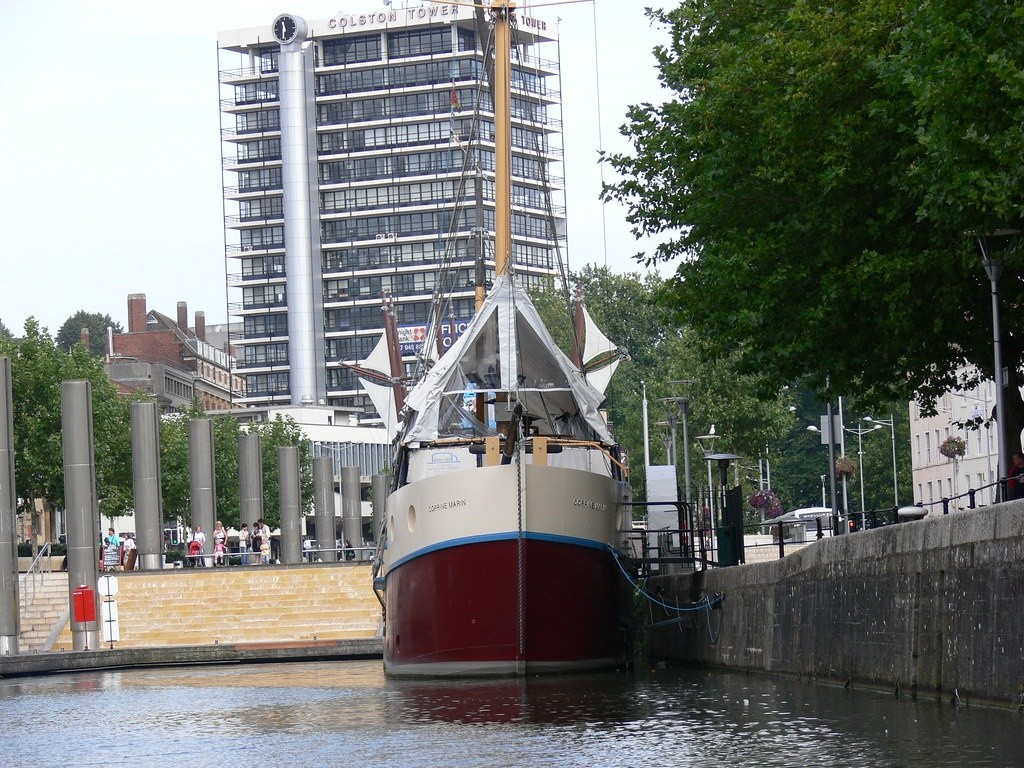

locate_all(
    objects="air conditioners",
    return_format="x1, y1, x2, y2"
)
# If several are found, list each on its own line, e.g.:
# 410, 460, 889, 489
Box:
244, 246, 254, 252
252, 413, 262, 422
375, 233, 385, 240
387, 232, 397, 238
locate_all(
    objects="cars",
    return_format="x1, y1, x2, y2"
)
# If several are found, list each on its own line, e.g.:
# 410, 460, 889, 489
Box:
303, 538, 378, 561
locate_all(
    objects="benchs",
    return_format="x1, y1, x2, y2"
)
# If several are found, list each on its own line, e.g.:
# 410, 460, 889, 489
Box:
302, 547, 376, 564
183, 552, 262, 568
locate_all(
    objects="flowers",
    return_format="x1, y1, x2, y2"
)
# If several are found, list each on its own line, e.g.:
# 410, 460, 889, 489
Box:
938, 436, 966, 459
746, 489, 774, 512
764, 495, 784, 519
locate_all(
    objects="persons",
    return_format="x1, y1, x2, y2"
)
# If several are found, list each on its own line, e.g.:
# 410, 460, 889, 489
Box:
238, 523, 250, 565
192, 526, 206, 567
122, 532, 137, 553
213, 521, 228, 566
336, 539, 356, 561
107, 528, 120, 547
1008, 452, 1024, 500
214, 539, 227, 565
251, 519, 271, 565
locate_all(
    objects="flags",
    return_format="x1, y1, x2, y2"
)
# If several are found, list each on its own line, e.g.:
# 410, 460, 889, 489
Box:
452, 79, 460, 110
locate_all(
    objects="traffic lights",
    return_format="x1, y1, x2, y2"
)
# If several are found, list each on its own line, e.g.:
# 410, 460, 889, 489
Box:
849, 521, 855, 533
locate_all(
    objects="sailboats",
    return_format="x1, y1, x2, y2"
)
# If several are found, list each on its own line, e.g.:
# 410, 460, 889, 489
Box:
367, 0, 715, 685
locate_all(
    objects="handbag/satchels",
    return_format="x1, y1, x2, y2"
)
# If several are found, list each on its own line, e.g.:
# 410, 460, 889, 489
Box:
346, 552, 353, 560
246, 539, 251, 547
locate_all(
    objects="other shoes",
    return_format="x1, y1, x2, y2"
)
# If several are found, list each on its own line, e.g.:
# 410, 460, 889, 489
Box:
180, 558, 273, 568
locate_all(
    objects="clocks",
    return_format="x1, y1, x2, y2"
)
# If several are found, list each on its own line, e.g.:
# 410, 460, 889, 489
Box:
273, 15, 296, 43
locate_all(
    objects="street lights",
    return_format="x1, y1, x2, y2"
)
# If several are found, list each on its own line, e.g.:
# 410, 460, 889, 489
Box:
657, 396, 688, 501
695, 435, 722, 566
962, 227, 1024, 503
842, 424, 883, 530
862, 413, 898, 507
667, 379, 698, 557
654, 420, 682, 466
